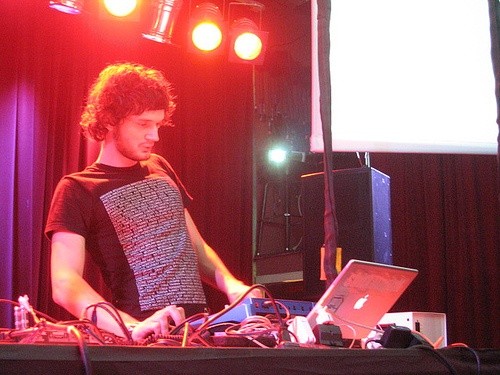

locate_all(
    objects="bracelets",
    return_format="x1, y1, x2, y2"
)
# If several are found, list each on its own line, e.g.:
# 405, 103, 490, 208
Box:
128, 321, 141, 336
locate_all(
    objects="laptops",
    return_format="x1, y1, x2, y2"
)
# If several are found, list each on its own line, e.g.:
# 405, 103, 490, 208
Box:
292, 259, 419, 338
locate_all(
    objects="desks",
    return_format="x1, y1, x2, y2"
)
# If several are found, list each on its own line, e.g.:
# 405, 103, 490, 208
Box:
0, 337, 500, 375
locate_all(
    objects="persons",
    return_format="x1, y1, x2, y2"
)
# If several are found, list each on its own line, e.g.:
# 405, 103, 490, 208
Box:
44, 60, 265, 341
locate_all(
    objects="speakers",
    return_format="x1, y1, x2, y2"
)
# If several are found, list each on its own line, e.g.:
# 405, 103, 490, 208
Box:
301, 167, 393, 298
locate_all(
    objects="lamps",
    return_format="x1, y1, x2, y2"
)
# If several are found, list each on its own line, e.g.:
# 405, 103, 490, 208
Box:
98, 1, 139, 22
227, 17, 269, 66
186, 2, 227, 58
48, 0, 85, 15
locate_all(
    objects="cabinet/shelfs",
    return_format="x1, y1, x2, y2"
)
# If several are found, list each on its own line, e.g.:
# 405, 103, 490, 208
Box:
253, 176, 303, 261
378, 311, 449, 349
299, 165, 393, 300
